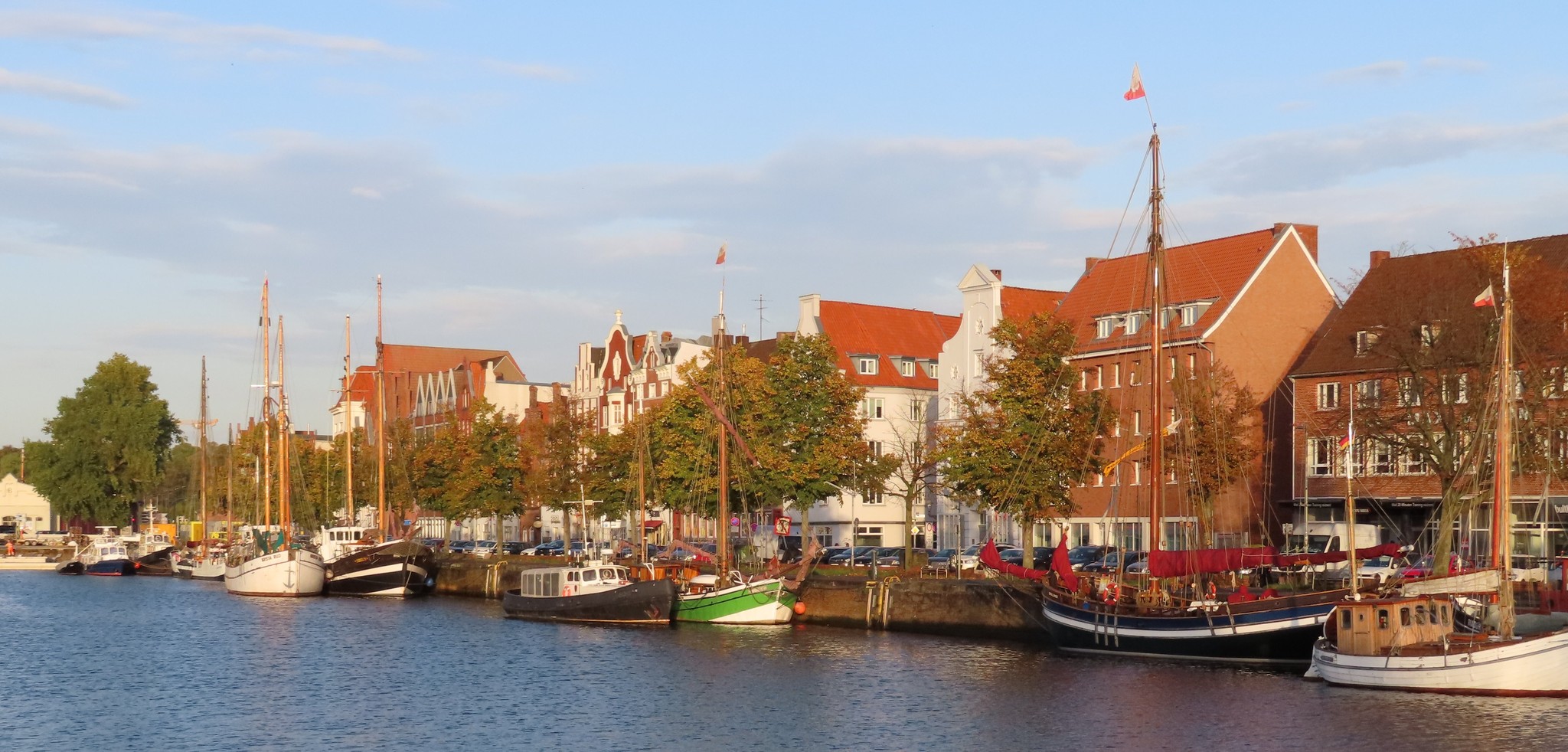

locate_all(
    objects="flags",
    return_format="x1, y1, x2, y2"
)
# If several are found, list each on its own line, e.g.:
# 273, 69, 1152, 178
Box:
1474, 285, 1495, 307
715, 243, 727, 264
1124, 64, 1145, 101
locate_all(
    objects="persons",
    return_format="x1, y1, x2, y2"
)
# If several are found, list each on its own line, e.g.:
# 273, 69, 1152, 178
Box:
835, 543, 849, 547
20, 527, 24, 534
5, 541, 14, 556
471, 537, 474, 541
579, 538, 593, 542
1259, 567, 1287, 588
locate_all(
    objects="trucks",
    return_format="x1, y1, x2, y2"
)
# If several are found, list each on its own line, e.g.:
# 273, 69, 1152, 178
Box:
1271, 521, 1387, 576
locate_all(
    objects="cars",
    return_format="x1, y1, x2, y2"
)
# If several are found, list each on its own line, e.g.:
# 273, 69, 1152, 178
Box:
1125, 556, 1149, 573
927, 549, 966, 573
414, 538, 737, 564
1503, 555, 1562, 591
1356, 553, 1474, 578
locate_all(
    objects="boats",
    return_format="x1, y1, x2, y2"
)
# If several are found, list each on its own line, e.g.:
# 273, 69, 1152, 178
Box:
503, 484, 677, 632
309, 524, 369, 565
55, 495, 173, 575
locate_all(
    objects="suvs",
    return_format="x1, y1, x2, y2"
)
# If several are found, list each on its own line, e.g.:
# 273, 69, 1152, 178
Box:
788, 546, 937, 571
958, 542, 1147, 574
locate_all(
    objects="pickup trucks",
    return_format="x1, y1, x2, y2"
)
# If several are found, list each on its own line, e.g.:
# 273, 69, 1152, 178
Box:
0, 524, 20, 545
19, 530, 70, 546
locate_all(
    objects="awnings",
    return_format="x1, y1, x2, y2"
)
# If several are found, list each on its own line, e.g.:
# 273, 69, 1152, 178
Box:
636, 521, 664, 530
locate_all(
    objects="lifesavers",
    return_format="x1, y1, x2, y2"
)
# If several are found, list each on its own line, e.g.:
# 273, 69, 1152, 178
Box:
1102, 583, 1120, 606
619, 581, 631, 584
563, 586, 571, 597
1205, 582, 1216, 600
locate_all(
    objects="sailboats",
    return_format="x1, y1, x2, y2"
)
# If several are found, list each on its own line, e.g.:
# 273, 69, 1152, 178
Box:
1304, 227, 1568, 704
978, 124, 1385, 664
224, 279, 324, 597
619, 412, 702, 595
322, 272, 439, 599
671, 270, 826, 629
170, 356, 228, 581
